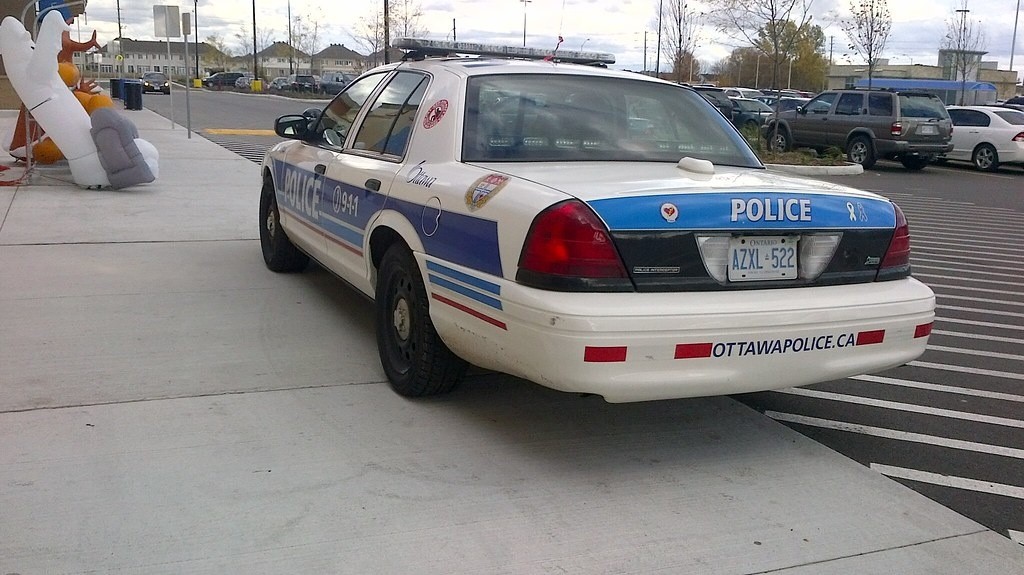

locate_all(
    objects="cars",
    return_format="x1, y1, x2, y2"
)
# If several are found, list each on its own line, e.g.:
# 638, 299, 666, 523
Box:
201, 70, 246, 88
268, 71, 360, 96
934, 94, 1024, 172
138, 70, 170, 96
256, 36, 937, 404
479, 80, 832, 140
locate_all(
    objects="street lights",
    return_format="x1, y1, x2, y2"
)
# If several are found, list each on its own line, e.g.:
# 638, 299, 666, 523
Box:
953, 9, 970, 82
520, 0, 532, 47
787, 55, 801, 89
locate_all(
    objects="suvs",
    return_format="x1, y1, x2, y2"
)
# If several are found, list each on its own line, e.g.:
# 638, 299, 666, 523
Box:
761, 85, 956, 171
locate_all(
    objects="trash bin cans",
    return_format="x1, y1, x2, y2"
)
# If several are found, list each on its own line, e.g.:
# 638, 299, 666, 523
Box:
124, 82, 143, 111
110, 78, 119, 98
